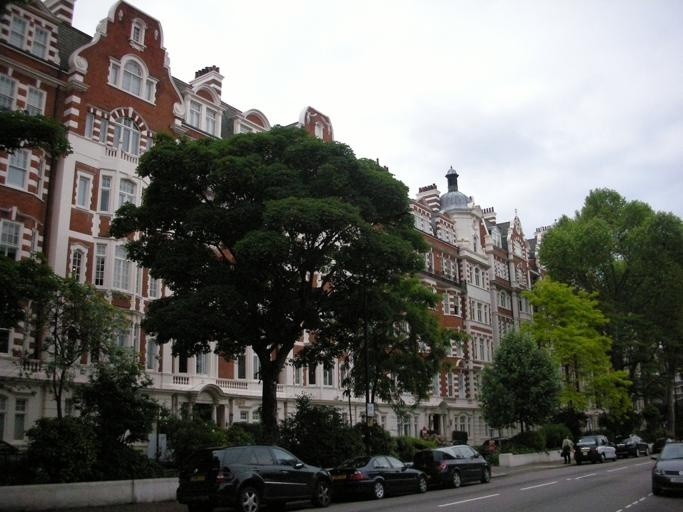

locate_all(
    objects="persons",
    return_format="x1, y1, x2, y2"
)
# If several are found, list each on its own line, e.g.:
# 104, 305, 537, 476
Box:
420, 426, 448, 446
562, 436, 575, 464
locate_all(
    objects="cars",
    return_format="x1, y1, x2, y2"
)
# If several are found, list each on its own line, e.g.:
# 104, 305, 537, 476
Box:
574, 434, 683, 497
